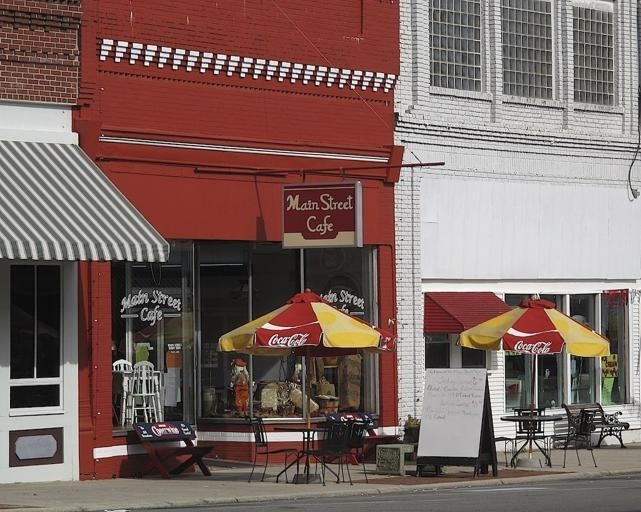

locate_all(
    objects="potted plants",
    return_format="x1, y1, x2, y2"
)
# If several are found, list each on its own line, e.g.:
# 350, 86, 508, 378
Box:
405, 414, 421, 443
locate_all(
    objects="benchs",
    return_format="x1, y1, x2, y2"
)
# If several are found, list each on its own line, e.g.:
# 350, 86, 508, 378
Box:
561, 401, 630, 450
374, 444, 416, 477
131, 421, 217, 478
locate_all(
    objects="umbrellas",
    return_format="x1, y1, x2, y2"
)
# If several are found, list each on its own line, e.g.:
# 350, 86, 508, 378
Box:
217, 288, 398, 470
456, 292, 611, 460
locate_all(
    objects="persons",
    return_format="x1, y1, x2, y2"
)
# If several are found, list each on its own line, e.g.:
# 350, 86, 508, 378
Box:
230, 360, 255, 417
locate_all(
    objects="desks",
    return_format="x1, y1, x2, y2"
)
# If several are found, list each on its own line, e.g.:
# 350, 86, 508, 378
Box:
112, 370, 164, 423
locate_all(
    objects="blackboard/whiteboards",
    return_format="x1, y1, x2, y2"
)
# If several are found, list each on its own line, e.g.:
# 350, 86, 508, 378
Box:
416, 368, 496, 458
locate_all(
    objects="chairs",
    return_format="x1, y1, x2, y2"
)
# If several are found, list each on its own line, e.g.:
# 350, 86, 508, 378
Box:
495, 407, 599, 468
245, 413, 370, 485
113, 358, 158, 426
